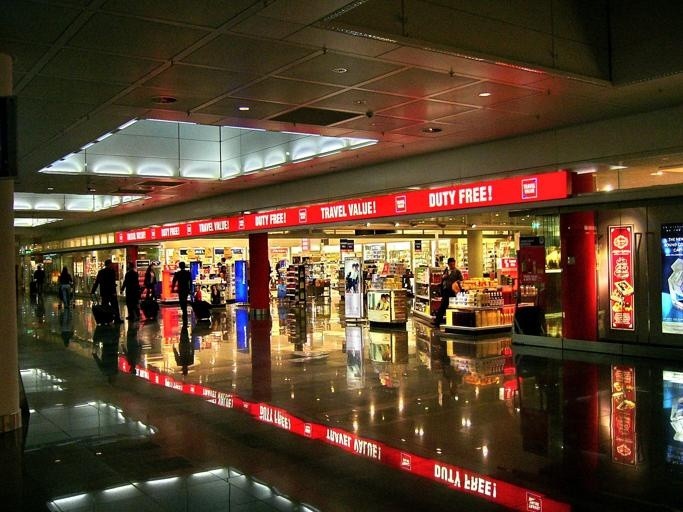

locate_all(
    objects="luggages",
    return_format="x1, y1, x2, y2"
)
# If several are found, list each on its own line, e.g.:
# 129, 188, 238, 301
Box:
190, 294, 210, 320
88, 292, 113, 326
140, 302, 157, 319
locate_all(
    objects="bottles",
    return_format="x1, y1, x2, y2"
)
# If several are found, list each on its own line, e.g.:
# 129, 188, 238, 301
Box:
481, 289, 504, 307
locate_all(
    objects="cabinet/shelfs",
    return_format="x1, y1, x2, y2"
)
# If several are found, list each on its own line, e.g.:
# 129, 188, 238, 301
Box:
162, 306, 513, 385
162, 257, 520, 333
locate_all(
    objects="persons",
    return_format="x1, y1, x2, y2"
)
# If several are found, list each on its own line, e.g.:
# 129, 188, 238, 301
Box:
60, 309, 74, 347
217, 257, 227, 277
436, 333, 459, 395
144, 264, 157, 297
33, 265, 45, 295
380, 294, 390, 311
375, 295, 390, 311
211, 285, 220, 305
91, 259, 124, 326
119, 262, 140, 321
173, 323, 194, 375
378, 344, 387, 361
268, 260, 273, 298
430, 257, 464, 329
58, 265, 74, 309
385, 344, 391, 362
348, 263, 359, 290
171, 261, 193, 323
33, 296, 46, 323
122, 320, 142, 374
90, 326, 120, 385
345, 263, 355, 292
